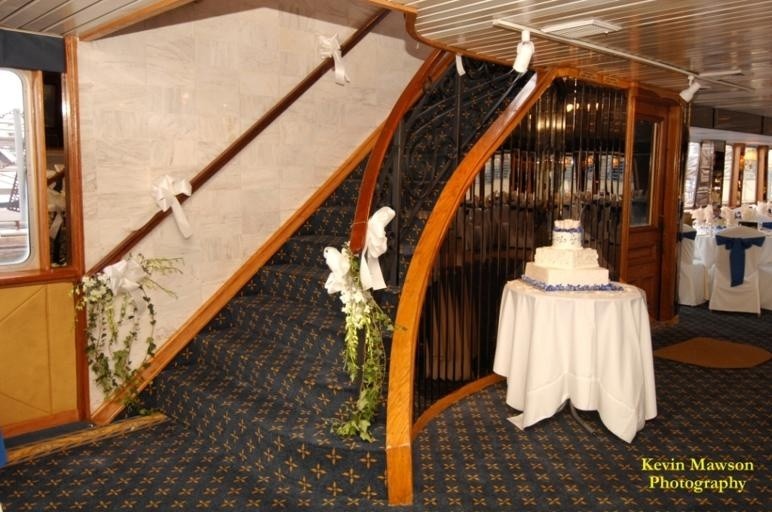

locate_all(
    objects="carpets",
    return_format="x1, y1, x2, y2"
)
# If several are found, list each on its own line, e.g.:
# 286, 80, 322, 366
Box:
651, 335, 772, 371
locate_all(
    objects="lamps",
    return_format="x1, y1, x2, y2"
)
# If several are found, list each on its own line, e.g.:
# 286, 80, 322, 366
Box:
679, 75, 701, 103
512, 27, 536, 73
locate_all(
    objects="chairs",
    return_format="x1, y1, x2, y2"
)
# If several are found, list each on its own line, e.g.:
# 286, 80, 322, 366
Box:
680, 201, 772, 317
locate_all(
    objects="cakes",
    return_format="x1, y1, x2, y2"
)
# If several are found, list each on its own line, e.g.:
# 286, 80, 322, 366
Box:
520, 218, 610, 291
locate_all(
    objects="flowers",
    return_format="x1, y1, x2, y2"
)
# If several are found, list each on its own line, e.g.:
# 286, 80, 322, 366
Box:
67, 251, 189, 418
316, 250, 398, 443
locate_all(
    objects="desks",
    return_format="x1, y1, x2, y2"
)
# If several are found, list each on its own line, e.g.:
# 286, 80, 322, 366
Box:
501, 279, 647, 436
